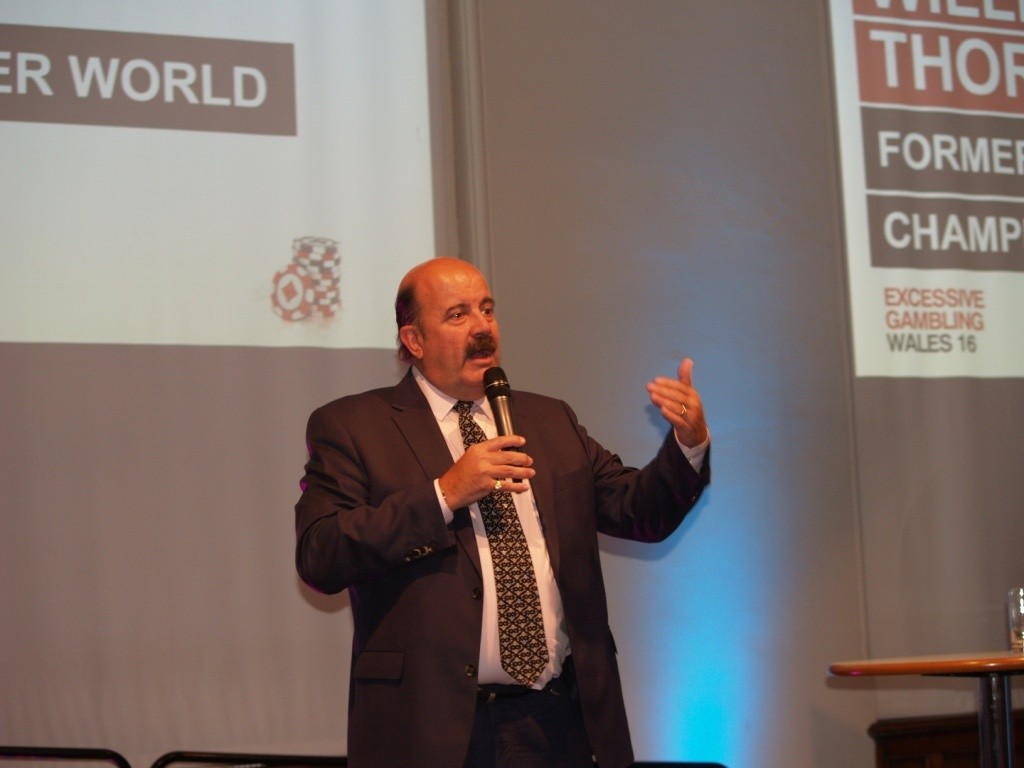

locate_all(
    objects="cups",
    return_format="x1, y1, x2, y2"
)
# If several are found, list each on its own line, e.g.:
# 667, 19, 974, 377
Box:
1008, 588, 1024, 654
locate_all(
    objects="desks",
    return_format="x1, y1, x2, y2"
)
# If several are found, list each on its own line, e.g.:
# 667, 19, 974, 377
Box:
828, 651, 1024, 768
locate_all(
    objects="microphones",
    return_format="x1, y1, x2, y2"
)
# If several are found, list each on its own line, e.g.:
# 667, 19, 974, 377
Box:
482, 366, 525, 495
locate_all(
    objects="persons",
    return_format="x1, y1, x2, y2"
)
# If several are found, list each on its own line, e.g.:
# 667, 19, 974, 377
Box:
295, 257, 709, 768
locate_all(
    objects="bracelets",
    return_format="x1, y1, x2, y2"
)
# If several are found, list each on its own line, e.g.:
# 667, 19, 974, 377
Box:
441, 491, 448, 503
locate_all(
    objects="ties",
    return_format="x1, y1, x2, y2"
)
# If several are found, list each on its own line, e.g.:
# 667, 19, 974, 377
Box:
454, 400, 549, 688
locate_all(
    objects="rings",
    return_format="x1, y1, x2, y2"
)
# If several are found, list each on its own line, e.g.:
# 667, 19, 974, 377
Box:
494, 480, 502, 493
680, 405, 688, 417
